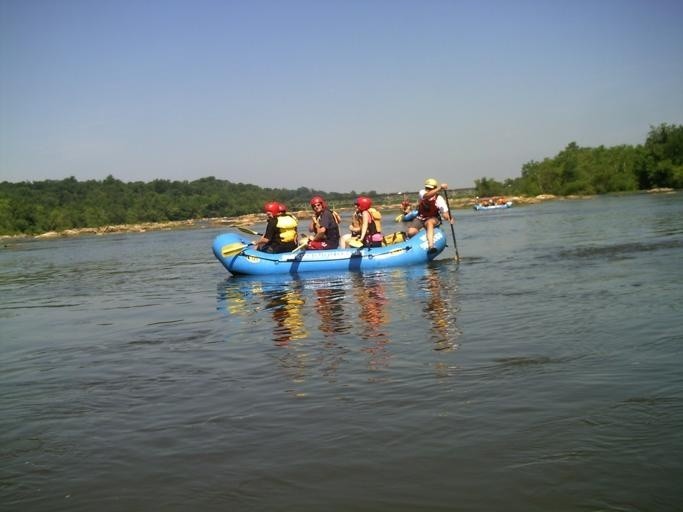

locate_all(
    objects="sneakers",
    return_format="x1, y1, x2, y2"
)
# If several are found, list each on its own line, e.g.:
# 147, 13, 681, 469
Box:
430, 248, 437, 254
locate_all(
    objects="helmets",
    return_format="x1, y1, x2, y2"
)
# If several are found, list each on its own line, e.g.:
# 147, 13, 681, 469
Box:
265, 202, 286, 217
357, 196, 372, 210
311, 197, 325, 209
425, 179, 437, 189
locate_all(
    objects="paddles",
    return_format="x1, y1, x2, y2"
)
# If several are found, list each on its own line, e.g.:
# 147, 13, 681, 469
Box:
221, 241, 256, 258
291, 238, 309, 254
237, 227, 263, 235
348, 239, 364, 248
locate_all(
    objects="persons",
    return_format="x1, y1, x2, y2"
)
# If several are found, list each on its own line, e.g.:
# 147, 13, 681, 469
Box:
253, 201, 297, 254
339, 196, 382, 248
299, 196, 339, 250
349, 197, 362, 236
474, 193, 507, 207
277, 203, 297, 225
400, 193, 409, 214
407, 179, 455, 255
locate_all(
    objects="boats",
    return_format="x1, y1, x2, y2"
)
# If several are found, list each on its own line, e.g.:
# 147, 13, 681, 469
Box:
209, 224, 448, 278
473, 201, 514, 211
